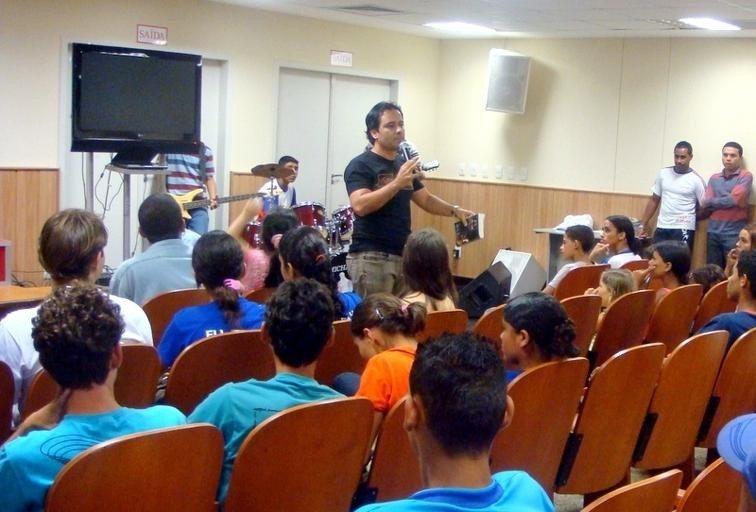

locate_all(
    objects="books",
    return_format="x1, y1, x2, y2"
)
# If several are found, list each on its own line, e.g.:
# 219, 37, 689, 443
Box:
455, 213, 485, 246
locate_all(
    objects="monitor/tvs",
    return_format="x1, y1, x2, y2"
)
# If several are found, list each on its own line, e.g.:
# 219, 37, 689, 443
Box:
71, 42, 203, 169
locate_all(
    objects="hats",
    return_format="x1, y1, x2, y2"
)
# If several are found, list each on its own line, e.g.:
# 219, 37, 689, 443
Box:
716, 413, 755, 502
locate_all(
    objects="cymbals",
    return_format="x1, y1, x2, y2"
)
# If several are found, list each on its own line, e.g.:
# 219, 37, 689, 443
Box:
250, 162, 295, 179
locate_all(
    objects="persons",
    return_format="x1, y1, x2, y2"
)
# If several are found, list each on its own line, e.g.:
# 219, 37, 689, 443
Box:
186, 275, 349, 505
0, 206, 156, 431
349, 290, 426, 468
105, 191, 206, 311
642, 141, 707, 249
158, 139, 221, 238
701, 141, 754, 270
344, 100, 476, 296
500, 291, 579, 380
154, 228, 271, 375
224, 195, 300, 295
401, 227, 459, 311
354, 332, 553, 511
256, 155, 299, 214
277, 226, 364, 320
0, 280, 187, 510
544, 215, 756, 352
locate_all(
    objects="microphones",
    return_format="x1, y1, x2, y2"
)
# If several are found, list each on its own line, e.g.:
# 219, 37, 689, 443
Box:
399, 141, 423, 192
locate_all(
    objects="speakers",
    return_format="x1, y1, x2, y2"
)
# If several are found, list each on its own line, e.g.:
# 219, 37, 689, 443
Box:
484, 48, 532, 114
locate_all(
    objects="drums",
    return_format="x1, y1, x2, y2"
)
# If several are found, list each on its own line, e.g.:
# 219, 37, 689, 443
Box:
332, 206, 358, 234
324, 220, 345, 251
240, 219, 266, 252
287, 201, 328, 241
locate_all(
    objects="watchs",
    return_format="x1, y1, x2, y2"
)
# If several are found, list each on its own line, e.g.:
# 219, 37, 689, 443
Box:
452, 204, 460, 216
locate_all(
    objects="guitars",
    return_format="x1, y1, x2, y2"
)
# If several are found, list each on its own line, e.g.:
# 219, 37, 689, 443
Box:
164, 186, 285, 222
